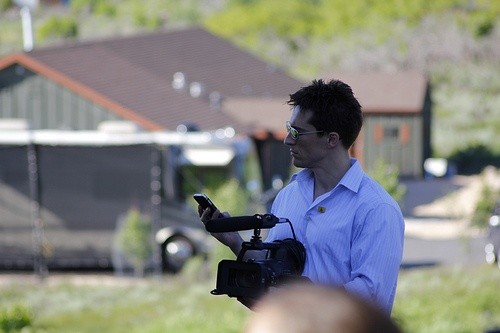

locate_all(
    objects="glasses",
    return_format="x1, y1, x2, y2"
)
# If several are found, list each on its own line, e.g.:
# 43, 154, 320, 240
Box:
285, 120, 325, 138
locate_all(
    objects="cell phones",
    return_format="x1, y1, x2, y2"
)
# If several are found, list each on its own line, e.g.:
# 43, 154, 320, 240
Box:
194, 194, 224, 219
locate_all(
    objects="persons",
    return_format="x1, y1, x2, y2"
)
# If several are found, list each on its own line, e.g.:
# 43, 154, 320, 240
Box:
199, 79, 404, 320
243, 280, 407, 333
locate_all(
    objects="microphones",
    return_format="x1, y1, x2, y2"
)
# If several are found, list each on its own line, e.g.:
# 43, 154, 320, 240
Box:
205, 214, 289, 233
